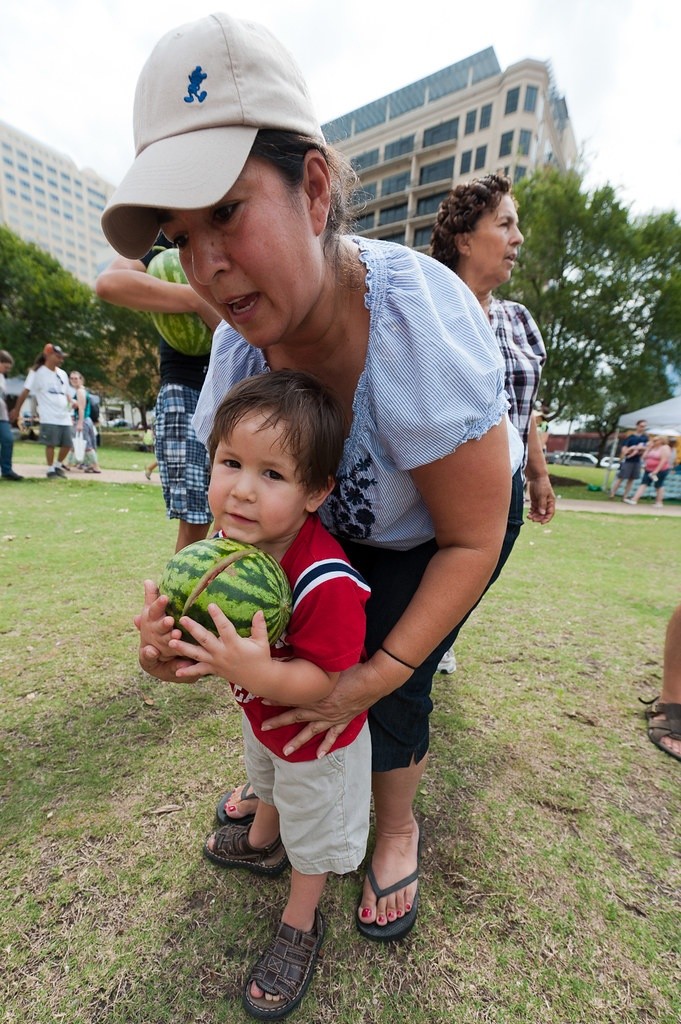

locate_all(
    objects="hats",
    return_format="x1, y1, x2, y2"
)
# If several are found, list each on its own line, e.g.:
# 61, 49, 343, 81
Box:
43, 343, 69, 357
101, 10, 326, 261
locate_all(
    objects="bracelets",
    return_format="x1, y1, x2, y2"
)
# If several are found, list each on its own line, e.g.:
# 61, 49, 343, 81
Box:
383, 646, 417, 674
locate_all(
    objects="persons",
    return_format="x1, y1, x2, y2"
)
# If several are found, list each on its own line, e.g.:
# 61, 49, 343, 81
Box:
609, 421, 651, 501
0, 340, 100, 481
151, 370, 371, 1015
623, 435, 672, 509
639, 601, 681, 758
99, 14, 523, 946
432, 174, 555, 677
97, 235, 226, 553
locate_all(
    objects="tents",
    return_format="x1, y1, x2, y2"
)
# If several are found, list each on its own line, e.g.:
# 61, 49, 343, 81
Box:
604, 395, 681, 495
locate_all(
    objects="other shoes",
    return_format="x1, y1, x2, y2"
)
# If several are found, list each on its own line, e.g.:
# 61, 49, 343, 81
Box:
47, 471, 57, 479
2, 471, 24, 481
652, 502, 662, 508
55, 467, 67, 479
623, 498, 636, 505
84, 466, 102, 472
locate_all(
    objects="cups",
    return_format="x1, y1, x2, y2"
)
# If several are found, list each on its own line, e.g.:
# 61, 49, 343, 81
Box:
649, 472, 658, 481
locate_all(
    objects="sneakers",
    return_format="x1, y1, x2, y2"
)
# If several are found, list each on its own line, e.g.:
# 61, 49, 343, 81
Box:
436, 645, 457, 673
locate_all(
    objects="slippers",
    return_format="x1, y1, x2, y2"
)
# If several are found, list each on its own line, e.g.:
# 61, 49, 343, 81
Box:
354, 830, 423, 943
214, 781, 261, 825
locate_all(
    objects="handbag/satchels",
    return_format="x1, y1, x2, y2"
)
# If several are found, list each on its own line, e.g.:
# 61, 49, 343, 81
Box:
88, 392, 100, 422
72, 429, 88, 463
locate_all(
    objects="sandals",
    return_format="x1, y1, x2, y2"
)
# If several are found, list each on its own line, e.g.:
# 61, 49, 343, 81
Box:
646, 694, 681, 761
204, 822, 289, 876
242, 907, 325, 1019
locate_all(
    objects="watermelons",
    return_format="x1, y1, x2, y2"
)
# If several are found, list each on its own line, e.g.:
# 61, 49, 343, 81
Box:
158, 537, 292, 646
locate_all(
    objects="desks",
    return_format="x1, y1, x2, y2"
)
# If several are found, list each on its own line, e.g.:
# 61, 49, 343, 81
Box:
610, 470, 681, 500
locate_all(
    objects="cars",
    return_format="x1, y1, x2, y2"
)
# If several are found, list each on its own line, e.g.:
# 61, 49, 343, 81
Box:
546, 450, 621, 470
